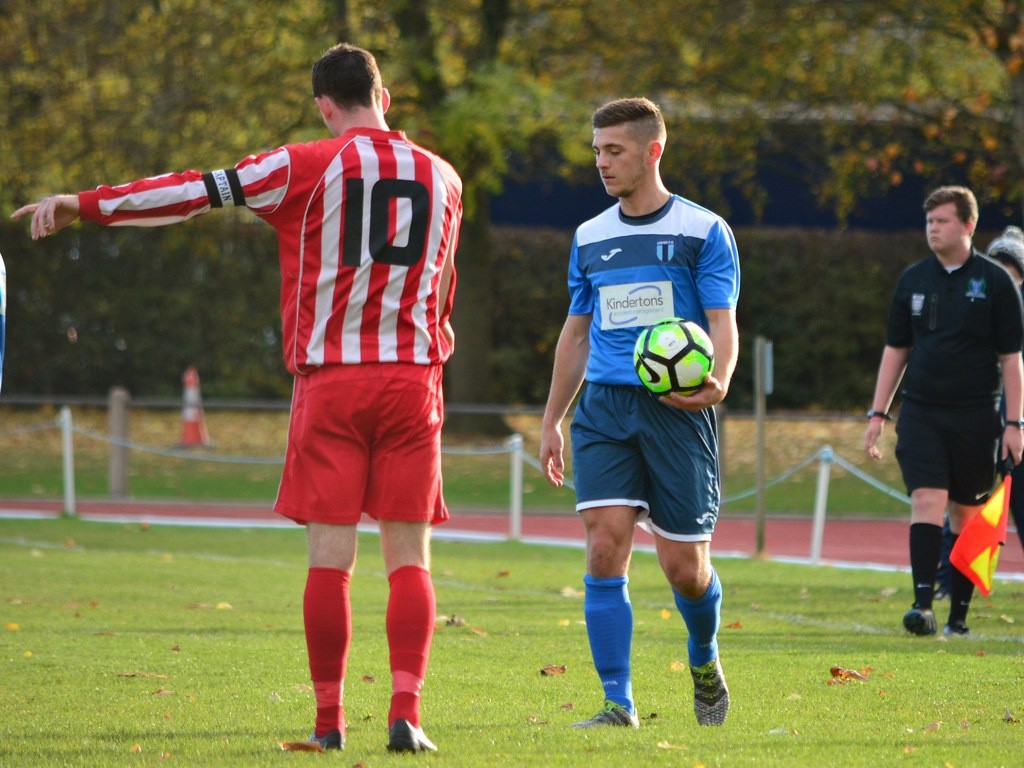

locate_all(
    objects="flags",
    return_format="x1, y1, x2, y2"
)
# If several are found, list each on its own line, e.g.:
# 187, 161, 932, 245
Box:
950, 475, 1011, 597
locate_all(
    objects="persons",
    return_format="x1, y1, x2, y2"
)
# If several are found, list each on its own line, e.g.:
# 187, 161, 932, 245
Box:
12, 40, 464, 753
929, 225, 1024, 600
865, 186, 1024, 637
539, 98, 739, 725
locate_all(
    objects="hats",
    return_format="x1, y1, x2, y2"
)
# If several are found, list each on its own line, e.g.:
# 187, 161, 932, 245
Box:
986, 225, 1024, 270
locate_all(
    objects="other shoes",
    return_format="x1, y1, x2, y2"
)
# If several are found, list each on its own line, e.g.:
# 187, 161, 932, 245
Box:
902, 601, 937, 635
944, 620, 971, 639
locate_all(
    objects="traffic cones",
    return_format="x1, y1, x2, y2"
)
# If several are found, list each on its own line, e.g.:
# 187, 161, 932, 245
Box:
170, 365, 222, 453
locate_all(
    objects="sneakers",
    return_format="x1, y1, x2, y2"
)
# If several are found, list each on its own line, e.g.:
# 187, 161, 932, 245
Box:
687, 652, 730, 728
307, 728, 346, 753
386, 717, 438, 754
569, 703, 642, 732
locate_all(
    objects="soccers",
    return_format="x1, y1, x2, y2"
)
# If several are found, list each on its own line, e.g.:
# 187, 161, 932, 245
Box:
633, 318, 716, 400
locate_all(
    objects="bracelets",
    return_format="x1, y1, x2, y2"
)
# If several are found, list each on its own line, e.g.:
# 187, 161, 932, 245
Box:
1007, 421, 1024, 426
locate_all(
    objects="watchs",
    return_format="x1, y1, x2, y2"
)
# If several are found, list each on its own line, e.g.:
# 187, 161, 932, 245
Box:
866, 410, 890, 420
